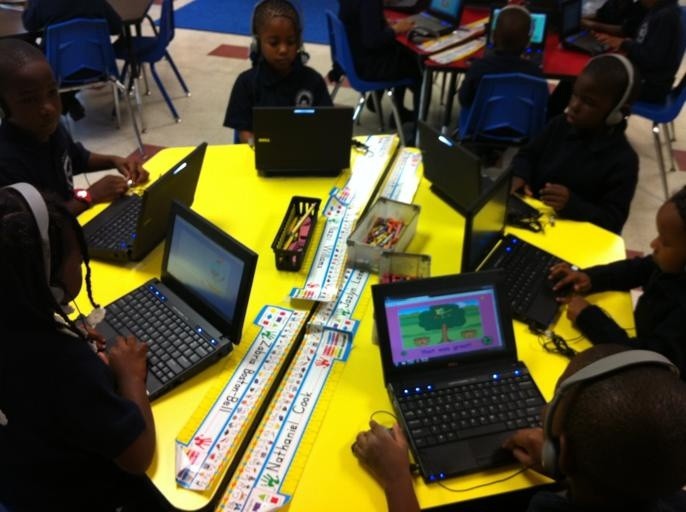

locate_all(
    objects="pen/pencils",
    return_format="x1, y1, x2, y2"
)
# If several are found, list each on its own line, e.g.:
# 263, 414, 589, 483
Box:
288, 216, 298, 231
292, 202, 317, 234
282, 236, 294, 250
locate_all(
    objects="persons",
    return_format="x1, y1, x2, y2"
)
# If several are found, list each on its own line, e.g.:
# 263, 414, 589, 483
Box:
21, 0, 122, 123
1, 38, 150, 215
352, 343, 686, 512
509, 52, 639, 235
458, 9, 543, 166
546, 1, 680, 125
338, 0, 414, 129
548, 183, 686, 383
1, 182, 156, 512
222, 0, 335, 143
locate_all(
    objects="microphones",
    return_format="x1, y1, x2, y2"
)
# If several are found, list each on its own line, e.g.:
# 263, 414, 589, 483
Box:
564, 106, 569, 114
59, 305, 75, 314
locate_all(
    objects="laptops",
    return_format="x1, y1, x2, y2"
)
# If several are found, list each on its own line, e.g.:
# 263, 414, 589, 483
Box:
484, 6, 548, 73
461, 168, 576, 333
371, 267, 547, 484
557, 0, 614, 55
393, 0, 467, 37
82, 142, 208, 263
417, 117, 539, 224
72, 199, 258, 402
252, 106, 354, 176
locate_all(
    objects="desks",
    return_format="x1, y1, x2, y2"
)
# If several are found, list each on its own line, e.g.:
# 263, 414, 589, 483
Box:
58, 133, 640, 512
0, 0, 31, 39
381, 1, 626, 149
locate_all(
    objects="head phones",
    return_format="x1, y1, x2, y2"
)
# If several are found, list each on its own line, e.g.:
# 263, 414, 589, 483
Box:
492, 4, 535, 37
2, 181, 65, 305
540, 349, 681, 480
250, 0, 303, 54
584, 53, 635, 127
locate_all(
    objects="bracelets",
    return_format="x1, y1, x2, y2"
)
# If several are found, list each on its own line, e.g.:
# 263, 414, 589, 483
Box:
70, 188, 92, 208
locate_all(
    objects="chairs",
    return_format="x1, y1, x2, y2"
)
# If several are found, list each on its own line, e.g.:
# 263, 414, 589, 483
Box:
626, 75, 686, 197
110, 0, 190, 123
326, 8, 407, 148
456, 74, 550, 143
